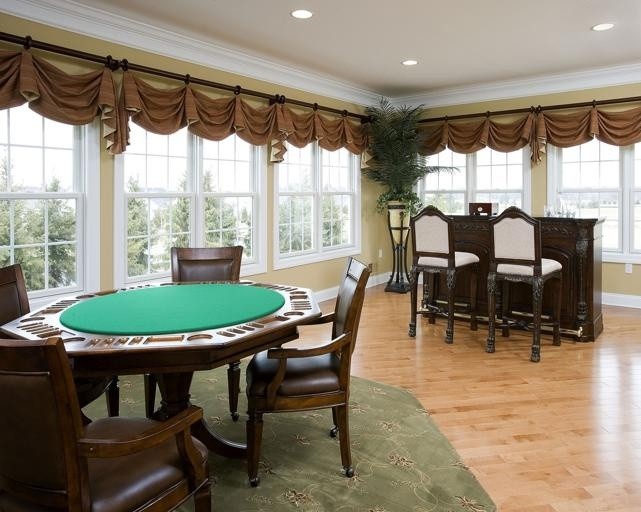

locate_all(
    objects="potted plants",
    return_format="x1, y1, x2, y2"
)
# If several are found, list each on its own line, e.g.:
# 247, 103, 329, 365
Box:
357, 90, 461, 247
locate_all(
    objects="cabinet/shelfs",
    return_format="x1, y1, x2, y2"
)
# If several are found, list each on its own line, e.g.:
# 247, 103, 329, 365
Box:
422, 210, 608, 345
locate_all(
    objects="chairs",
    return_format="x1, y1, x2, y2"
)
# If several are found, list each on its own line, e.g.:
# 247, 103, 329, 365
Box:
0, 245, 373, 511
408, 202, 569, 364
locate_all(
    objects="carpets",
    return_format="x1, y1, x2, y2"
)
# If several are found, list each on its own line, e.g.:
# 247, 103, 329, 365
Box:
83, 360, 500, 512
